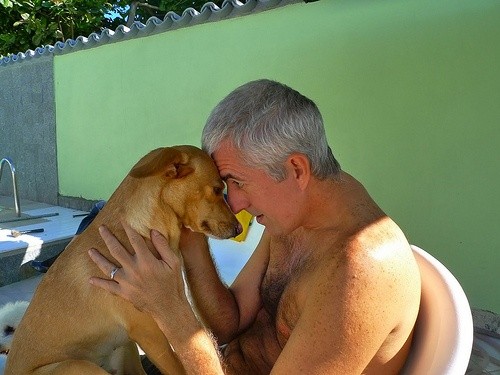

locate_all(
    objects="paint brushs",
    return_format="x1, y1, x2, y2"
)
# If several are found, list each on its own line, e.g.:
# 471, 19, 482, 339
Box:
11, 228, 44, 238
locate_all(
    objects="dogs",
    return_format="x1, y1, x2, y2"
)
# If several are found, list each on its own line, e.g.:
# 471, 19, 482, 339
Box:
3, 145, 243, 375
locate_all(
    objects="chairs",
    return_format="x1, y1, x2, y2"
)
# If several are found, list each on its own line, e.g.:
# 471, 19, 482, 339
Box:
401, 243, 474, 375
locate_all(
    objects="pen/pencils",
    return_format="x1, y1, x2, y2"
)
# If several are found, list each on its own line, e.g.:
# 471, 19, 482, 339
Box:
73, 214, 89, 218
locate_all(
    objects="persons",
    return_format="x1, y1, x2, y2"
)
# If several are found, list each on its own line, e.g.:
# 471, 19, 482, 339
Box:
87, 76, 421, 375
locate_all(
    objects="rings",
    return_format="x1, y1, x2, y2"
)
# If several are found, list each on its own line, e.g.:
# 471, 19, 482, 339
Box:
110, 266, 122, 280
